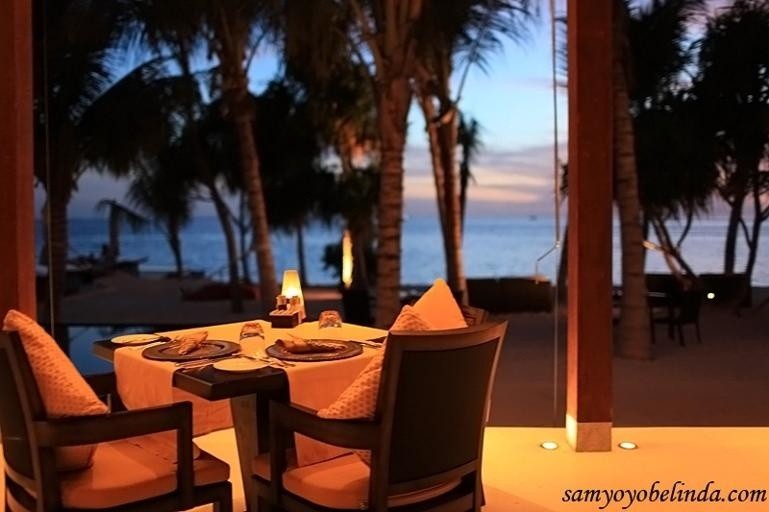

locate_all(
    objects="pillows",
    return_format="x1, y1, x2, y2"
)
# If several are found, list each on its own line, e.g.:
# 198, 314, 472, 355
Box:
1, 308, 106, 475
318, 304, 435, 468
415, 277, 469, 330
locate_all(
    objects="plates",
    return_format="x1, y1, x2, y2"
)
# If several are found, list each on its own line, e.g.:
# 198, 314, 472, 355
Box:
110, 333, 169, 346
265, 339, 363, 361
214, 357, 270, 374
141, 339, 242, 361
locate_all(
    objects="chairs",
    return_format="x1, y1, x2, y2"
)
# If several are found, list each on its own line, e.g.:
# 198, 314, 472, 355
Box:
672, 291, 703, 346
251, 320, 508, 512
649, 295, 676, 343
0, 329, 232, 512
412, 299, 487, 327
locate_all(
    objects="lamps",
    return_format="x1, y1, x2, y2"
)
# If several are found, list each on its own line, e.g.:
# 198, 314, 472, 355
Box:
280, 270, 305, 305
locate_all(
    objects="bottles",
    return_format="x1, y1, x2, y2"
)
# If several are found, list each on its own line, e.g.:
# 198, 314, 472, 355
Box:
275, 295, 287, 309
290, 295, 301, 325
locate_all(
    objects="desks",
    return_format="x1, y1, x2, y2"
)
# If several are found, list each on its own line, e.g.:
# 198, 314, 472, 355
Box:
608, 289, 676, 323
